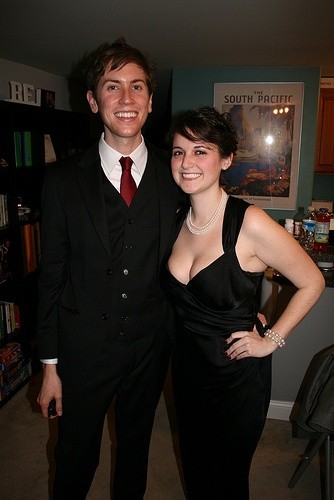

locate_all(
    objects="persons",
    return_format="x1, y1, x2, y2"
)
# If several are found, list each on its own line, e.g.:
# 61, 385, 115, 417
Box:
159, 105, 325, 500
35, 36, 186, 500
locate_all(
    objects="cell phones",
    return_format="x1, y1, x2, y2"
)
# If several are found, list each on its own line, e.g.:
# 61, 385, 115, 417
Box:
48, 399, 57, 419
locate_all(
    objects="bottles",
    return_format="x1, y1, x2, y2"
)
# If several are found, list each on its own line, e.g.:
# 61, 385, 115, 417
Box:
299, 207, 330, 250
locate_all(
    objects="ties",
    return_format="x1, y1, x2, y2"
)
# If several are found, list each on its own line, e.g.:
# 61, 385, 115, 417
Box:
119, 156, 137, 207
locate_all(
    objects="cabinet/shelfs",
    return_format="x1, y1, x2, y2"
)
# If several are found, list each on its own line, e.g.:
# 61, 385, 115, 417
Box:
0, 95, 91, 411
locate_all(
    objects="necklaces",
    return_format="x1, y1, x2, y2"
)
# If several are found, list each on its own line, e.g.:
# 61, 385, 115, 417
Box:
185, 189, 227, 235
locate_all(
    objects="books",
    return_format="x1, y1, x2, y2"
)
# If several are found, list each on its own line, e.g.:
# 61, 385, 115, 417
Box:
0, 195, 9, 227
0, 342, 29, 400
24, 221, 42, 273
0, 300, 21, 338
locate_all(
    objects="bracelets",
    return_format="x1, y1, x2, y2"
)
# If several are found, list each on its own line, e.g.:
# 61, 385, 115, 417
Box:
264, 329, 285, 348
